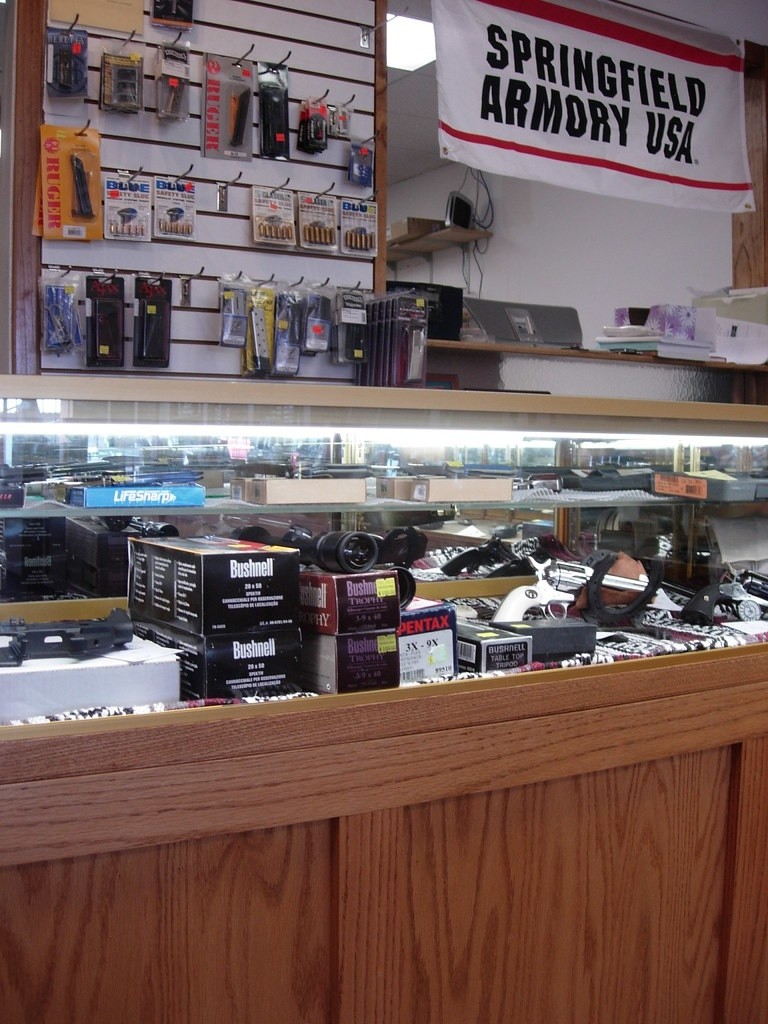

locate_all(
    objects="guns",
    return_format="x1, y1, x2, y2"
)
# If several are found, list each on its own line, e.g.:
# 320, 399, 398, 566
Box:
0, 607, 136, 668
435, 531, 767, 629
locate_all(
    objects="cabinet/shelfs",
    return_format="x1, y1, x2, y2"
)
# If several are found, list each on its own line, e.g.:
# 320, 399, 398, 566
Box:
0, 373, 768, 1024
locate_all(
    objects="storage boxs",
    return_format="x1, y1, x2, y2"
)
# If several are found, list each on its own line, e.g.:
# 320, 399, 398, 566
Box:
55, 482, 205, 508
0, 518, 140, 602
489, 617, 597, 663
300, 568, 400, 635
651, 471, 756, 504
126, 534, 300, 636
306, 633, 401, 694
397, 599, 459, 684
129, 609, 306, 699
410, 479, 513, 503
195, 470, 223, 488
231, 477, 366, 505
456, 619, 533, 674
